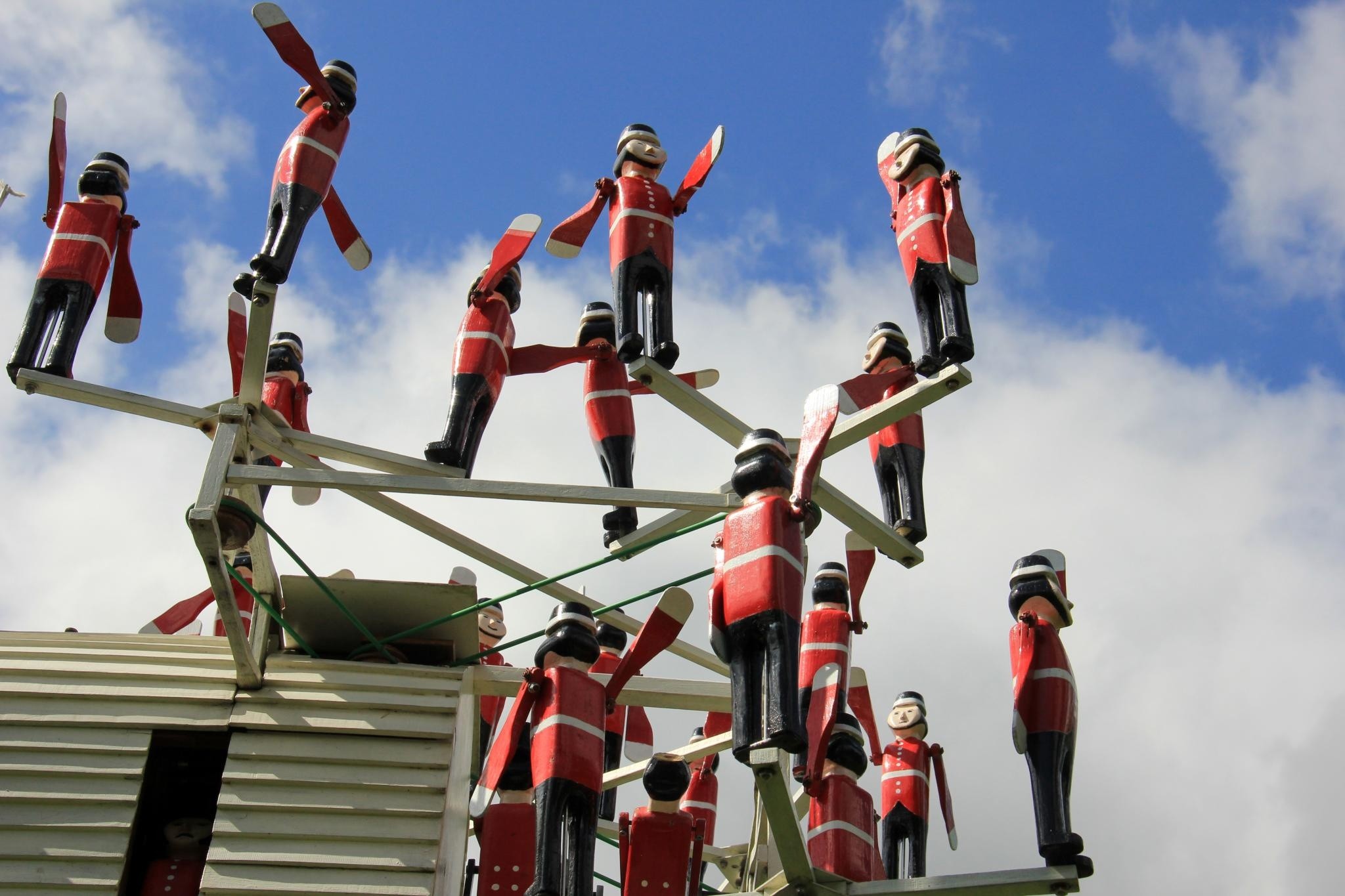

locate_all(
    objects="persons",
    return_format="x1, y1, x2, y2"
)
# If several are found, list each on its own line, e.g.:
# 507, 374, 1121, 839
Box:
1008, 549, 1093, 879
138, 292, 355, 638
232, 1, 371, 303
707, 384, 841, 769
424, 123, 726, 548
836, 320, 927, 561
5, 91, 143, 388
459, 561, 958, 896
877, 129, 979, 378
